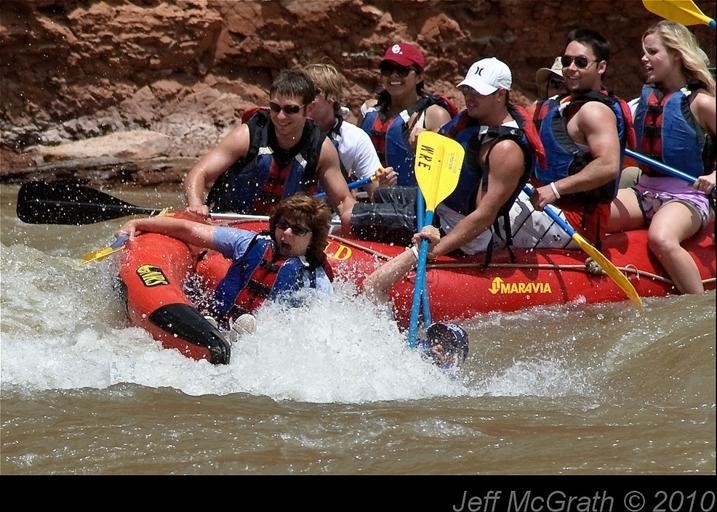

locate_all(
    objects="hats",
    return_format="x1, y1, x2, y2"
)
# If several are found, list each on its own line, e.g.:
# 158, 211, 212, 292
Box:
536, 56, 564, 87
426, 320, 469, 359
379, 43, 425, 69
456, 58, 513, 96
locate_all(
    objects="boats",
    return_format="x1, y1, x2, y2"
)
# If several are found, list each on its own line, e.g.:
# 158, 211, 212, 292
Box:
112, 209, 716, 365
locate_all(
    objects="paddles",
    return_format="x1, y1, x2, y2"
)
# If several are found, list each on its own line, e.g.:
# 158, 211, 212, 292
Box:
16, 181, 342, 225
407, 131, 465, 349
78, 234, 129, 264
643, 1, 716, 28
523, 184, 643, 308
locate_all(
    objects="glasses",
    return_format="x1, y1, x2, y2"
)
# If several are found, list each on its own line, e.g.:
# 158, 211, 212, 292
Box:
268, 101, 305, 114
461, 86, 499, 97
549, 76, 564, 89
561, 55, 597, 68
380, 64, 415, 76
275, 219, 311, 237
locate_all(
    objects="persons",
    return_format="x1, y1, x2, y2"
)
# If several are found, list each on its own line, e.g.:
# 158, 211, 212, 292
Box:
114, 22, 717, 370
